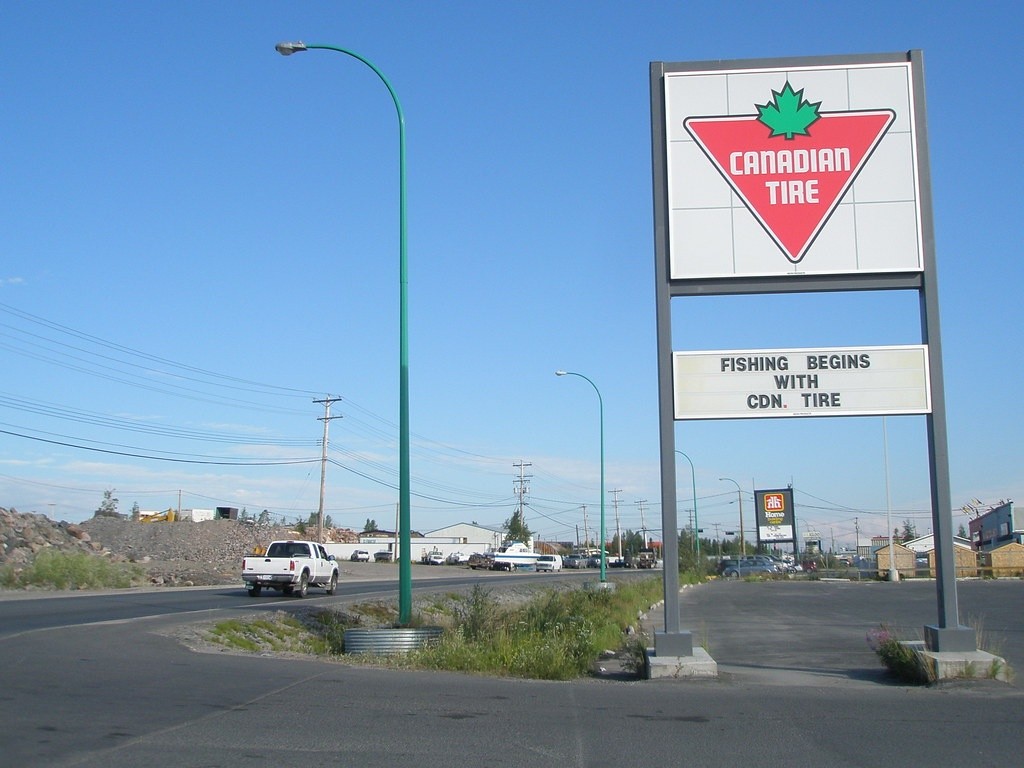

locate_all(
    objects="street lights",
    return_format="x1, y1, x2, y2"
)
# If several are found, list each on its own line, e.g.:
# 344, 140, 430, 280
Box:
554, 370, 606, 584
277, 40, 413, 625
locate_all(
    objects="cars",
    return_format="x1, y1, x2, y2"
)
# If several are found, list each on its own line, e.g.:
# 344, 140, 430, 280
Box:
563, 554, 588, 568
714, 552, 855, 578
423, 551, 444, 565
349, 549, 371, 563
374, 551, 393, 563
445, 551, 469, 566
588, 550, 657, 571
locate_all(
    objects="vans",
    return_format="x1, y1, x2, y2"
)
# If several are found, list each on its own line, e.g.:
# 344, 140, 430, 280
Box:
535, 555, 563, 572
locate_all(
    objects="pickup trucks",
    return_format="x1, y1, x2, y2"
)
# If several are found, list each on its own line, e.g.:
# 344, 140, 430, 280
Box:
240, 539, 340, 599
